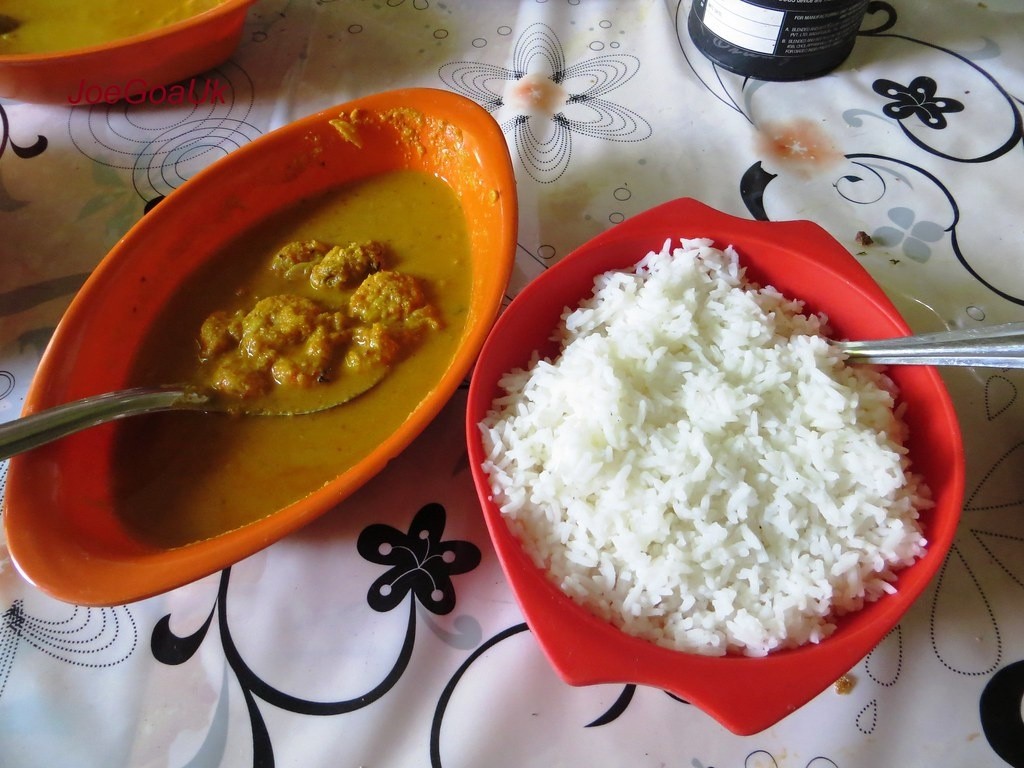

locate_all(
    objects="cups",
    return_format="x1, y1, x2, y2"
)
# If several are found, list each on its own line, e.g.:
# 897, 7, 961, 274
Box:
686, 0, 869, 82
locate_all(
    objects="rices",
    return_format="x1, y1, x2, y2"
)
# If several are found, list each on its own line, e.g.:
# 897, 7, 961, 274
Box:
477, 238, 940, 657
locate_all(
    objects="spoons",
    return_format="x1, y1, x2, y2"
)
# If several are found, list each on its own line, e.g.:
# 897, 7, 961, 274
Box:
0, 358, 388, 460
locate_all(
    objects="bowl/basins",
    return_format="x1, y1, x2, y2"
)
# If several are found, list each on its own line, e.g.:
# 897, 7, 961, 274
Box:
0, 90, 519, 607
459, 197, 966, 737
0, 0, 259, 108
